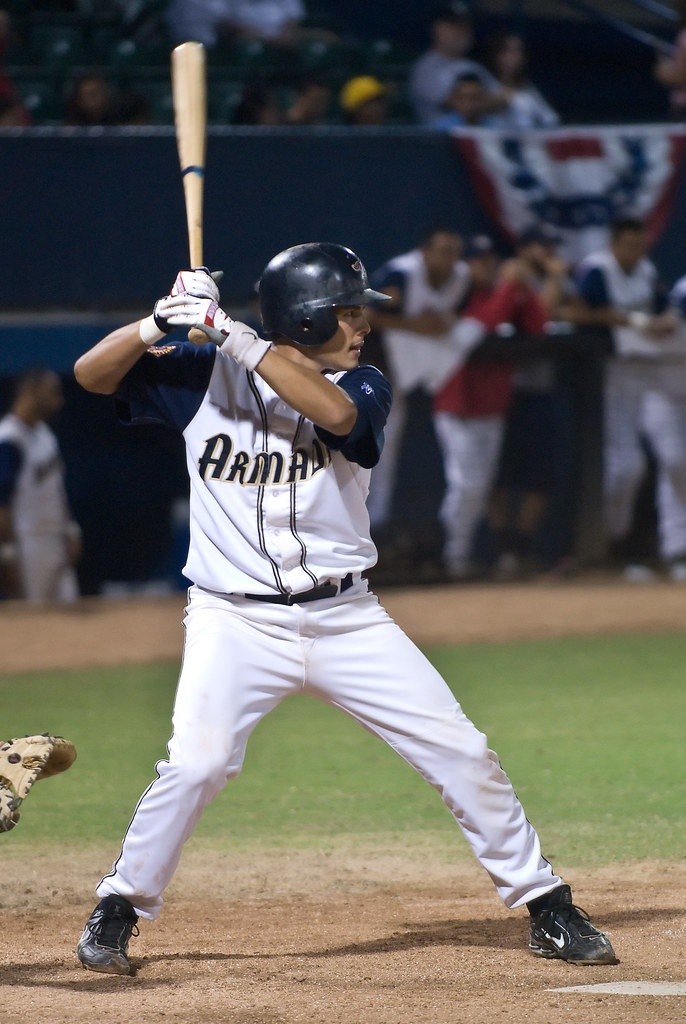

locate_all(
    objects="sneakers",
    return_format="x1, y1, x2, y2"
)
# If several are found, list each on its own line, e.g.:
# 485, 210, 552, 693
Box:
525, 884, 615, 964
76, 894, 140, 975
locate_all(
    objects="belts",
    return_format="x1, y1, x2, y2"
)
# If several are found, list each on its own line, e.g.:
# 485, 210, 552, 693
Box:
231, 573, 353, 606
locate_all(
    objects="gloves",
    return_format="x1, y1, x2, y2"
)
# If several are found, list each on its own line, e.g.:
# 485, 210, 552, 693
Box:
138, 271, 219, 345
156, 294, 273, 371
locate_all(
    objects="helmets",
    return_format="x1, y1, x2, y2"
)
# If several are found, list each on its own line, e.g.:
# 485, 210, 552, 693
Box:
522, 221, 563, 251
257, 241, 390, 346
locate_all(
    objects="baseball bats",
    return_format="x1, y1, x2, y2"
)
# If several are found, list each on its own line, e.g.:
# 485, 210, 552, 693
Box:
167, 40, 210, 343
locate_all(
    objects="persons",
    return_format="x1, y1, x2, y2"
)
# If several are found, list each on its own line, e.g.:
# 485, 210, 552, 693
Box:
0, 0, 685, 601
75, 243, 616, 975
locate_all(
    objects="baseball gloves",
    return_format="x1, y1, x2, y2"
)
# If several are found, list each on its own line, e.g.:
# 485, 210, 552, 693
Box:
0, 732, 78, 834
